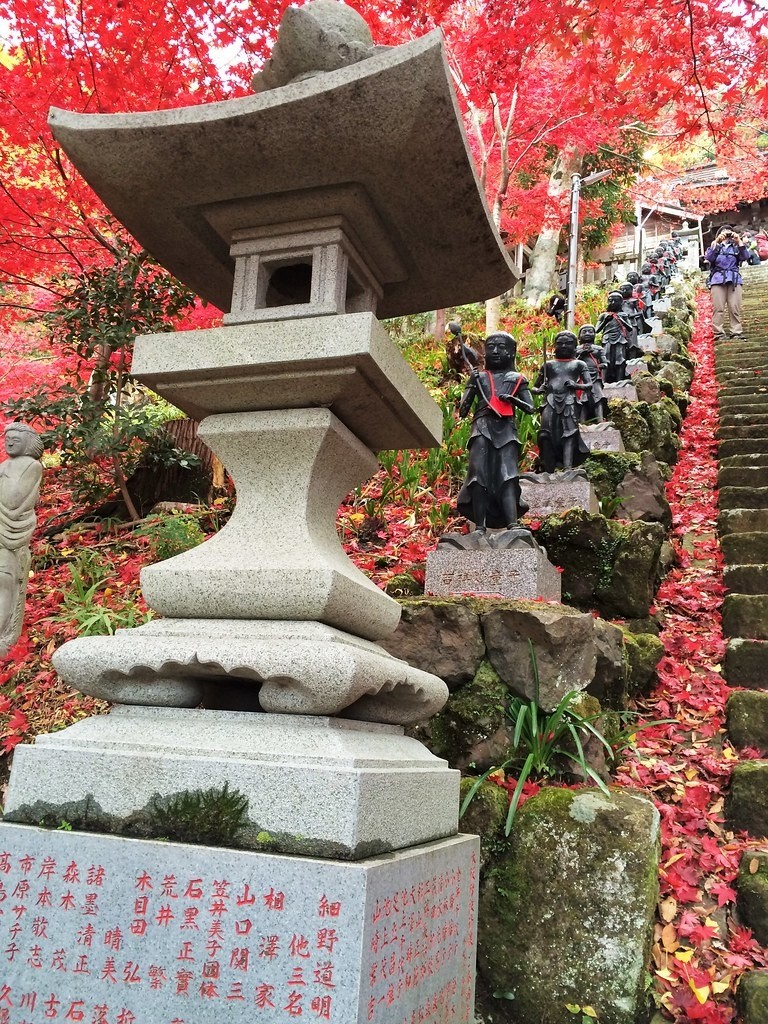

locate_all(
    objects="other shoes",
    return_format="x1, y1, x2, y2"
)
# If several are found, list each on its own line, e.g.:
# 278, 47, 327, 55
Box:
730, 335, 746, 339
713, 336, 725, 340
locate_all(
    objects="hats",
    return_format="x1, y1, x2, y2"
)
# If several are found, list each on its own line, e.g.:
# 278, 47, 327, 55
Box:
716, 224, 734, 237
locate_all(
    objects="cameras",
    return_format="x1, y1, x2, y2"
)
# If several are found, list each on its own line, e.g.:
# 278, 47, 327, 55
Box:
725, 233, 733, 239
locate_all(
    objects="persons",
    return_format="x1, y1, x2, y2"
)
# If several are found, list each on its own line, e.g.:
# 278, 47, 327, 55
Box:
0, 422, 44, 655
703, 225, 750, 342
449, 232, 683, 534
